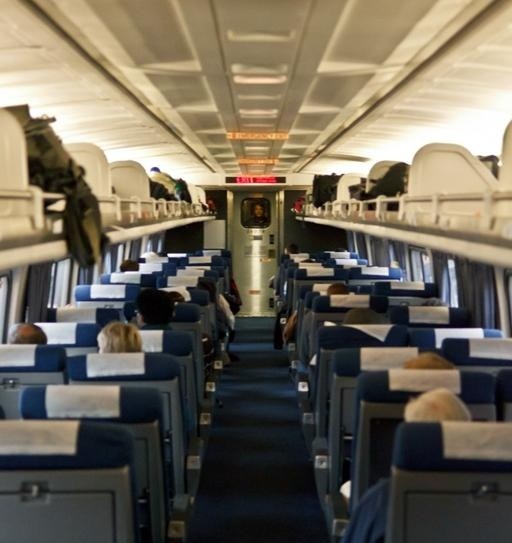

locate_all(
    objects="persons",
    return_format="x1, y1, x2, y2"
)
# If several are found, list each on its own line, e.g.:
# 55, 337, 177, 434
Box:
282, 309, 298, 346
243, 201, 268, 226
197, 280, 234, 340
134, 289, 174, 330
120, 260, 139, 272
8, 323, 47, 344
421, 297, 442, 306
269, 244, 299, 288
97, 322, 141, 352
338, 389, 471, 543
327, 283, 348, 296
400, 352, 452, 369
169, 291, 185, 302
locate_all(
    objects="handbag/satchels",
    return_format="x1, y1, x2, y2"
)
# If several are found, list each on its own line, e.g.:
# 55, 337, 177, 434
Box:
364, 154, 500, 209
4, 104, 108, 268
149, 179, 175, 199
289, 172, 341, 211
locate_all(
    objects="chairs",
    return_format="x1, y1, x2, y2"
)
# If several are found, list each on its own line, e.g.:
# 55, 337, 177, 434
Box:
1, 249, 233, 543
273, 250, 512, 542
